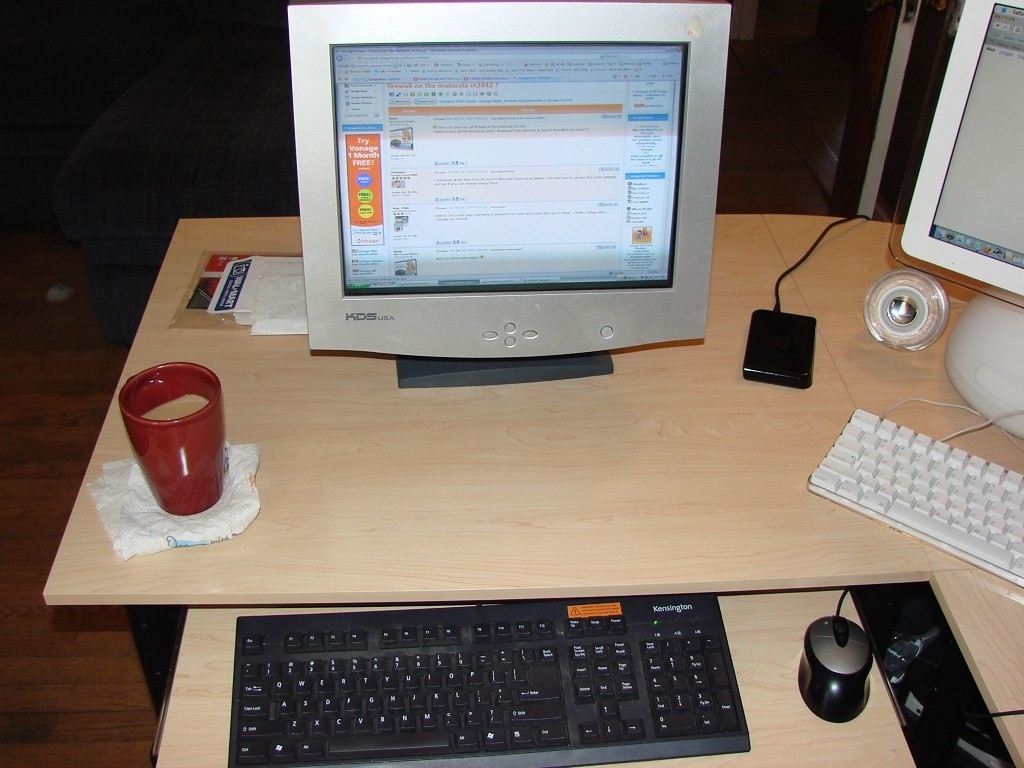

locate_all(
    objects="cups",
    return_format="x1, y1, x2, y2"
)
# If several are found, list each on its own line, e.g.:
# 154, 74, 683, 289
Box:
118, 362, 225, 516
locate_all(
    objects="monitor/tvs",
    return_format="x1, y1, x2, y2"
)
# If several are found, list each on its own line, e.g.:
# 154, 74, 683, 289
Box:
888, 0, 1024, 439
285, 0, 732, 388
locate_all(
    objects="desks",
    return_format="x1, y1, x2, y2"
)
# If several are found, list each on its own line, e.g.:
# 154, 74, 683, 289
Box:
42, 214, 1024, 768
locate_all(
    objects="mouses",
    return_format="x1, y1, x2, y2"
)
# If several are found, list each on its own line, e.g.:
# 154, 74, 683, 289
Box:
797, 615, 873, 724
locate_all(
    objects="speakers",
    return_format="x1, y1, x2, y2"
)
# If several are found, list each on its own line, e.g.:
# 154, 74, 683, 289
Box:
865, 268, 950, 351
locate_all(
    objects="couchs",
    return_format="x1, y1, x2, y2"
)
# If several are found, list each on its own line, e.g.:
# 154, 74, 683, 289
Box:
0, 0, 296, 341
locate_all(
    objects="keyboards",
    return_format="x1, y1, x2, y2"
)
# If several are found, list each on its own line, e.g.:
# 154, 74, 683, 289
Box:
807, 409, 1024, 589
228, 594, 752, 768
